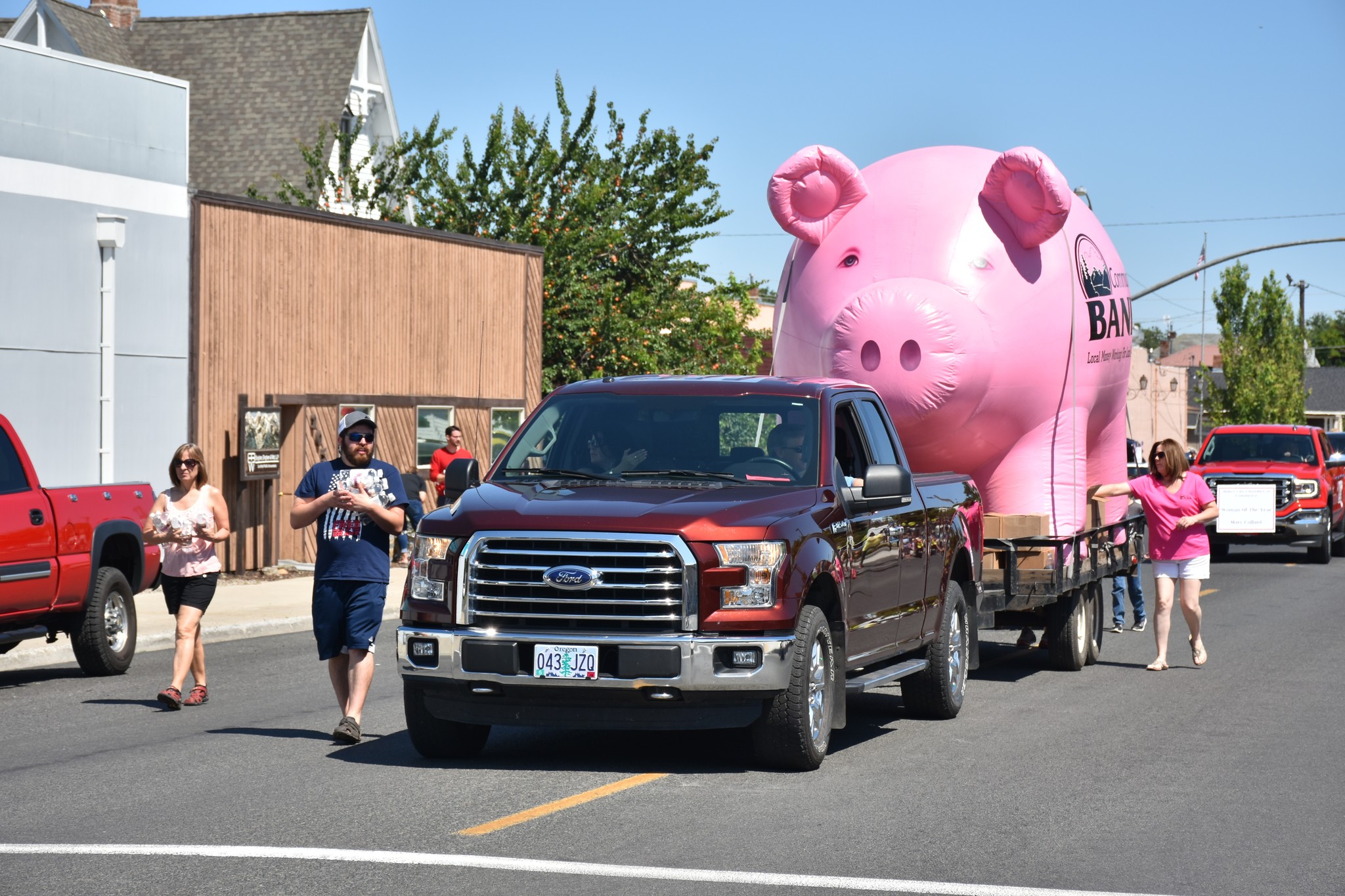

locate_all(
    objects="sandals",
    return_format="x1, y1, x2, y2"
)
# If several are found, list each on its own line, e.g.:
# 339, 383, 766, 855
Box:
184, 683, 209, 706
157, 686, 182, 710
332, 716, 361, 742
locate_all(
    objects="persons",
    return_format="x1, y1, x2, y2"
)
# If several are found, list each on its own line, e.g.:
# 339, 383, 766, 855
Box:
141, 442, 231, 712
1086, 438, 1218, 671
430, 426, 474, 507
762, 426, 865, 489
291, 410, 409, 740
571, 428, 648, 479
395, 466, 427, 565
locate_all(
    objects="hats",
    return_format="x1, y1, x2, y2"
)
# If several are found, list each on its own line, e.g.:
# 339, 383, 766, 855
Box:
338, 411, 377, 437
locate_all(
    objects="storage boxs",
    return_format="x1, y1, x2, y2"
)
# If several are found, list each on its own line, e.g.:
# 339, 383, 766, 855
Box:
1096, 497, 1108, 553
982, 552, 1005, 569
1084, 484, 1112, 554
983, 512, 1003, 552
1002, 512, 1050, 552
1005, 548, 1055, 570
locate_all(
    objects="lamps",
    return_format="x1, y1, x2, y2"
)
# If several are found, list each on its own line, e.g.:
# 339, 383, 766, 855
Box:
1150, 377, 1178, 401
1126, 375, 1148, 400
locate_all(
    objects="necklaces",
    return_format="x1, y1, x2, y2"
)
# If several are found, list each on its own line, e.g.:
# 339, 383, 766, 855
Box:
609, 461, 612, 472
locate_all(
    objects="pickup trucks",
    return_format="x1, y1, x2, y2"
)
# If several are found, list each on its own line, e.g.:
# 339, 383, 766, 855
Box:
394, 373, 987, 773
1127, 423, 1345, 565
0, 413, 165, 676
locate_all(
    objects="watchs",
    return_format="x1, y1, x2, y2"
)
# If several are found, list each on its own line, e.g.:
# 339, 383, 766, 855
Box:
608, 467, 614, 475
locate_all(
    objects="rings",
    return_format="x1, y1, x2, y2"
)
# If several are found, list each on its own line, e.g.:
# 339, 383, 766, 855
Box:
634, 455, 637, 459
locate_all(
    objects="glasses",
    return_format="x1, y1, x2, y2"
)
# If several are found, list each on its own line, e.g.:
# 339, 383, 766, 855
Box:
587, 438, 609, 449
172, 459, 200, 467
341, 432, 374, 442
779, 446, 803, 453
1152, 452, 1164, 459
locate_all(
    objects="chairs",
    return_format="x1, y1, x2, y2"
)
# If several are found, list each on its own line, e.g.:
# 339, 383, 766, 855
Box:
835, 427, 851, 476
1221, 445, 1243, 460
729, 447, 765, 462
602, 420, 654, 456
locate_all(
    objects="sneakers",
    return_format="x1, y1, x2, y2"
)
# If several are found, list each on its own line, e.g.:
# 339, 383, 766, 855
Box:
1131, 617, 1147, 632
1039, 630, 1050, 648
1016, 628, 1036, 646
1111, 622, 1123, 633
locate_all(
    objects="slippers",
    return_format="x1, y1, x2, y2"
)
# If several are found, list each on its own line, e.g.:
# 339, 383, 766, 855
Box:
1146, 661, 1169, 671
1188, 634, 1207, 665
